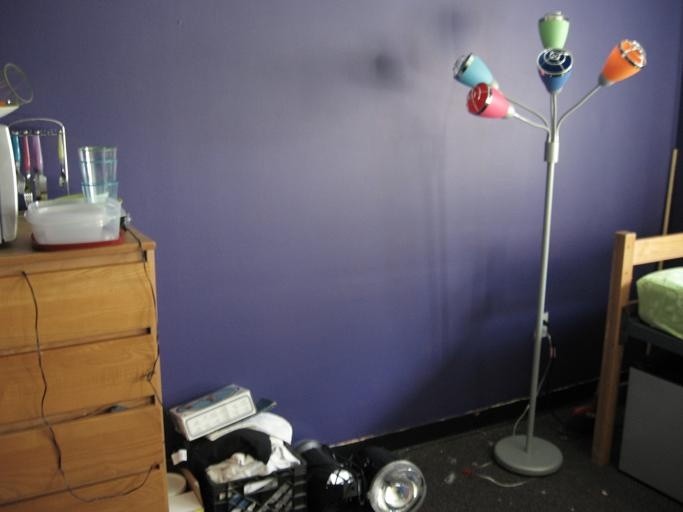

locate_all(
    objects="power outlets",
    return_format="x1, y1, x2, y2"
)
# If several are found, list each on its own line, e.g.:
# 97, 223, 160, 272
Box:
541, 313, 552, 339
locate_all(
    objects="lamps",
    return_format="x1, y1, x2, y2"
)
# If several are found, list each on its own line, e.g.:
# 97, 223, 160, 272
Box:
539, 10, 570, 50
537, 47, 574, 96
467, 83, 515, 120
453, 52, 499, 90
598, 38, 648, 88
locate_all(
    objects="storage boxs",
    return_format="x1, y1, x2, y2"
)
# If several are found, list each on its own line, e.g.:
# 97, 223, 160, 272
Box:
618, 365, 683, 504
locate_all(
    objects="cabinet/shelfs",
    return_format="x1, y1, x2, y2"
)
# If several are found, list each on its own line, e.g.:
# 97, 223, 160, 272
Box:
1, 207, 170, 512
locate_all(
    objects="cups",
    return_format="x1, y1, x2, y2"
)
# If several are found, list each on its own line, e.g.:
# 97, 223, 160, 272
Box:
78, 146, 117, 201
1, 62, 35, 106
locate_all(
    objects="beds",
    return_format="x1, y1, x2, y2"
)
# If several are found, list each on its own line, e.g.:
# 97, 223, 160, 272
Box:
592, 230, 683, 467
